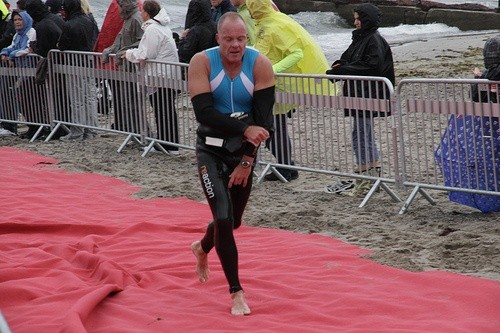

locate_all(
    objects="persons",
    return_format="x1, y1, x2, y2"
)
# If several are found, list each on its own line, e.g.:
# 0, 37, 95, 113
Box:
188, 12, 276, 316
121, 0, 182, 155
0, 0, 145, 140
325, 3, 395, 172
469, 37, 500, 101
178, 0, 339, 180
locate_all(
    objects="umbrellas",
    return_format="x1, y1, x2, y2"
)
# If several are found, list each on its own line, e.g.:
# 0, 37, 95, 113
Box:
435, 114, 500, 211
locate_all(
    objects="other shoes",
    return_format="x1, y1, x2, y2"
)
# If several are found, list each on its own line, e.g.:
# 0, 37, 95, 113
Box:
143, 145, 180, 155
60, 134, 85, 141
21, 127, 63, 141
325, 181, 355, 194
265, 161, 299, 181
0, 127, 18, 136
85, 131, 95, 138
356, 166, 382, 177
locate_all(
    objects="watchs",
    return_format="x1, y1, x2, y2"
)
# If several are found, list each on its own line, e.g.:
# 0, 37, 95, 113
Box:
240, 160, 254, 169
